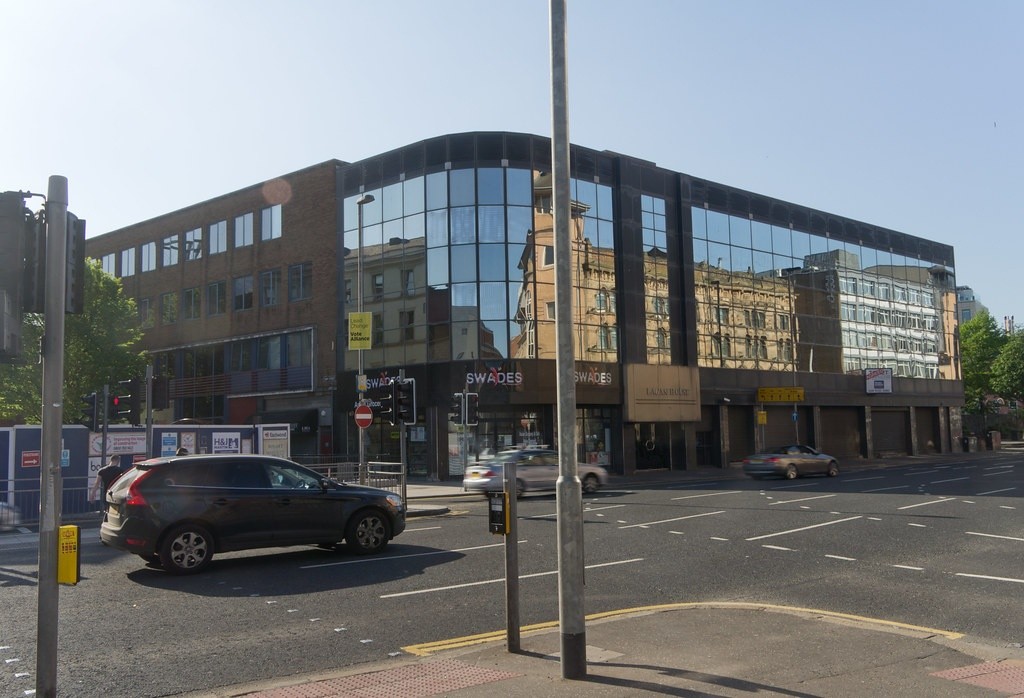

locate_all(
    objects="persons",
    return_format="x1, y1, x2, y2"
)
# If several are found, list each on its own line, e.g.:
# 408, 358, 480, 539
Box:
90, 455, 124, 545
176, 448, 188, 455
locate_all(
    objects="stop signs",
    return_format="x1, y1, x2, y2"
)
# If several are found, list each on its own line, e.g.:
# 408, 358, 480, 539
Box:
355, 405, 373, 428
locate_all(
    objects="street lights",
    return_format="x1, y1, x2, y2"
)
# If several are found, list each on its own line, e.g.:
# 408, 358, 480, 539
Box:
357, 195, 375, 486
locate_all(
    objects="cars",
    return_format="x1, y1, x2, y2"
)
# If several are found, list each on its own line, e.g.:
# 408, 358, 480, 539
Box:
0, 502, 24, 531
169, 417, 211, 425
743, 445, 839, 480
463, 445, 608, 500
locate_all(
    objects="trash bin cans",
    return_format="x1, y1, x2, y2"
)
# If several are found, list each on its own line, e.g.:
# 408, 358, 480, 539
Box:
962, 436, 978, 454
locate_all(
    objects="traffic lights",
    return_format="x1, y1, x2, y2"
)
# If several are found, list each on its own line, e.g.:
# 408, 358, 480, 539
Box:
450, 396, 461, 421
112, 396, 121, 418
397, 383, 409, 419
470, 397, 480, 423
118, 380, 131, 418
79, 397, 93, 429
378, 384, 392, 420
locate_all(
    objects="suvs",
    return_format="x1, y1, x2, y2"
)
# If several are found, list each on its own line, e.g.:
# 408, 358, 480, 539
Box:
99, 453, 404, 573
